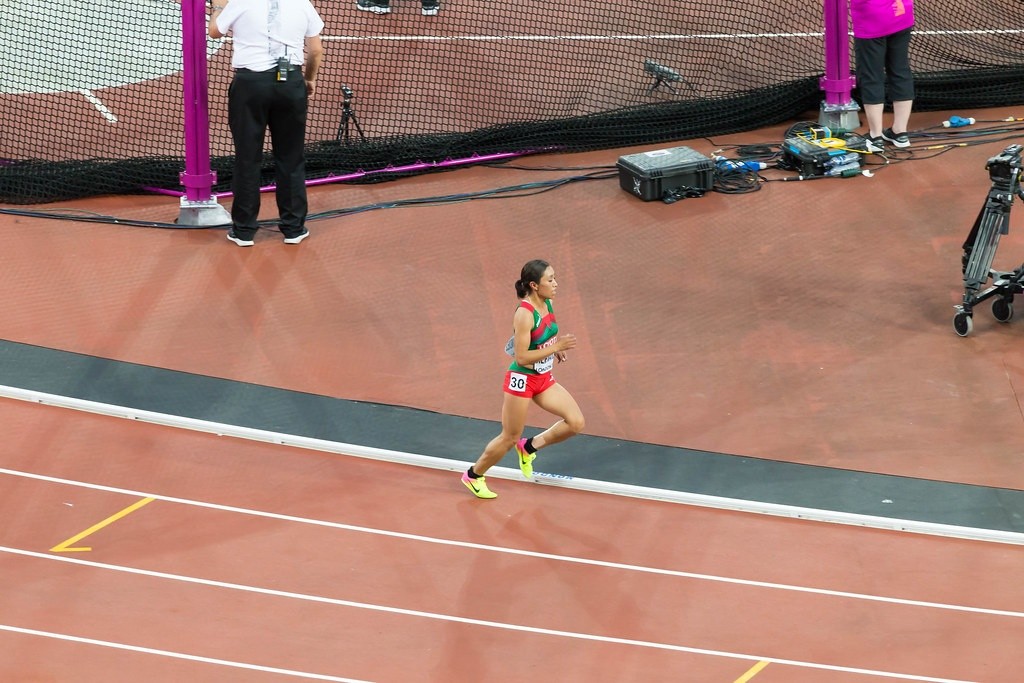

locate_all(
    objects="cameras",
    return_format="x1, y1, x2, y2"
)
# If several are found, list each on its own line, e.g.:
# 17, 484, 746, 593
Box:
341, 84, 354, 99
644, 60, 684, 85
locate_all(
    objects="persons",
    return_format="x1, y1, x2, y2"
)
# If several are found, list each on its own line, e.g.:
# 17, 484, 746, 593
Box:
356, 0, 440, 17
460, 260, 586, 499
207, 0, 326, 247
850, 0, 917, 153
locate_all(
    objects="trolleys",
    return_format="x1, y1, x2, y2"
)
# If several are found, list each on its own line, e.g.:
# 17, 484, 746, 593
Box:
954, 144, 1023, 337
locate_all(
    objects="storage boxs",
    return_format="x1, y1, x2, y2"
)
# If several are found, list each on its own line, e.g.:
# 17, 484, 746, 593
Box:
614, 146, 717, 203
780, 130, 868, 176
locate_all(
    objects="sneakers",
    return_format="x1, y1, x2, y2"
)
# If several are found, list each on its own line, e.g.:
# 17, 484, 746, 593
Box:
421, 1, 440, 16
514, 437, 537, 479
882, 125, 911, 148
227, 227, 256, 246
859, 132, 885, 153
460, 470, 498, 502
282, 225, 310, 245
355, 0, 391, 15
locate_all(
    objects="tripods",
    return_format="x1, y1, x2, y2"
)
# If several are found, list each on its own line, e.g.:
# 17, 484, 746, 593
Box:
335, 101, 364, 142
644, 77, 678, 96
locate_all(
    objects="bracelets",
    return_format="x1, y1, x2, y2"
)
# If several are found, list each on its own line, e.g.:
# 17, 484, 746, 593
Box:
210, 5, 225, 10
